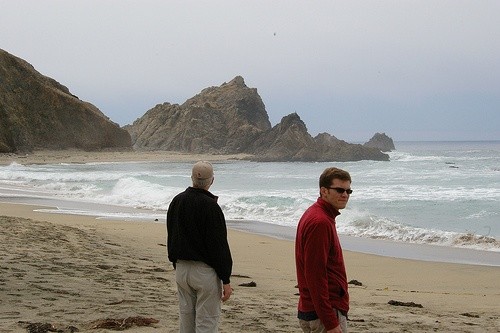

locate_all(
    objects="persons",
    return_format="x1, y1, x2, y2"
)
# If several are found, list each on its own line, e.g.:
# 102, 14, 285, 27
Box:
167, 161, 233, 333
296, 167, 352, 333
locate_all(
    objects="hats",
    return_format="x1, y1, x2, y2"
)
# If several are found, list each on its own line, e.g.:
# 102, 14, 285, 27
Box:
192, 161, 214, 179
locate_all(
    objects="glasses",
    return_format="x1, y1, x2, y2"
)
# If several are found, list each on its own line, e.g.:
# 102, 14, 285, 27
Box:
327, 188, 352, 194
